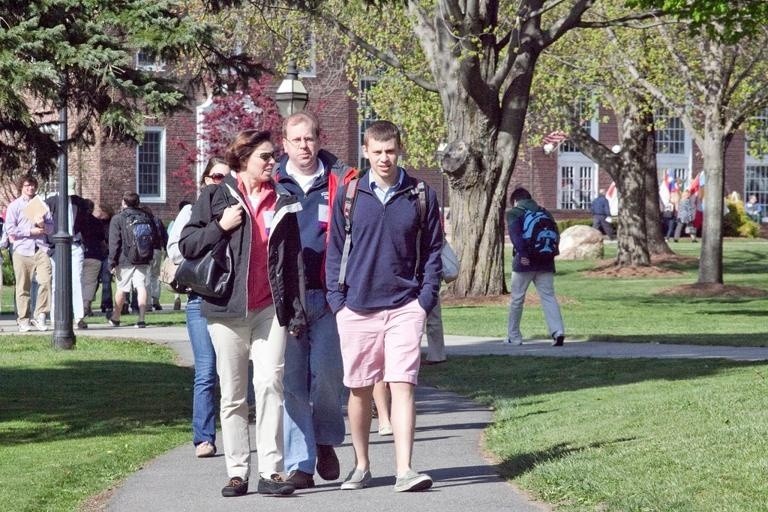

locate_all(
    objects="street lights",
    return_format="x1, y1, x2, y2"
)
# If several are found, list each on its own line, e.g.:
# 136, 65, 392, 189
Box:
274, 58, 307, 122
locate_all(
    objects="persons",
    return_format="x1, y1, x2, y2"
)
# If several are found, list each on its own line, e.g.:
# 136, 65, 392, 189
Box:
177, 128, 299, 497
504, 187, 564, 346
426, 211, 447, 362
0, 175, 190, 332
326, 120, 443, 492
591, 175, 762, 243
372, 379, 393, 436
167, 156, 231, 457
270, 111, 357, 488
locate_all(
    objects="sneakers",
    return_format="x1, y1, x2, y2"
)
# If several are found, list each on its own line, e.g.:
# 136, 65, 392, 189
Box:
379, 424, 392, 435
503, 338, 522, 346
16, 297, 181, 332
394, 471, 432, 492
316, 445, 340, 480
221, 476, 248, 497
258, 474, 294, 495
196, 440, 214, 457
287, 471, 315, 489
551, 330, 565, 346
340, 469, 371, 491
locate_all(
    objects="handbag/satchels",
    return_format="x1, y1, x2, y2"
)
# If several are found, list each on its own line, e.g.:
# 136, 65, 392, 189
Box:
158, 257, 192, 295
440, 240, 459, 283
170, 236, 234, 306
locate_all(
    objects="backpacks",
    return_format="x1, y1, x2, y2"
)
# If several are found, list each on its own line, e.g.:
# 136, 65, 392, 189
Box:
515, 206, 560, 259
120, 212, 155, 265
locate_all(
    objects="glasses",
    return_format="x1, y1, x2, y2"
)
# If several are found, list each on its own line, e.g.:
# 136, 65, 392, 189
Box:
259, 151, 276, 161
283, 137, 316, 147
208, 172, 224, 183
